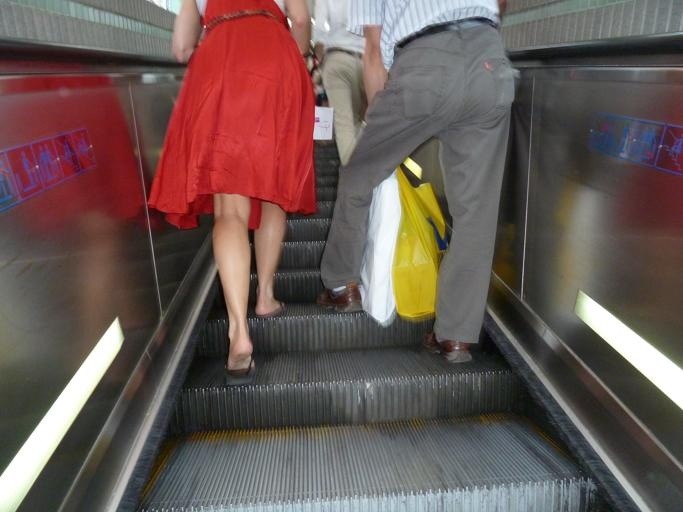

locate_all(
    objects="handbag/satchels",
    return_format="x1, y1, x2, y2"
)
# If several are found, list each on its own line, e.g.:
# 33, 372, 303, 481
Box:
357, 154, 445, 326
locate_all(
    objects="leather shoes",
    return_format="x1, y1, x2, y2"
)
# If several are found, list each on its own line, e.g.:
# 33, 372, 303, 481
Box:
423, 331, 473, 362
317, 282, 362, 313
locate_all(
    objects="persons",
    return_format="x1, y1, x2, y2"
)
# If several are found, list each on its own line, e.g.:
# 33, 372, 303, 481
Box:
312, 0, 366, 166
148, 0, 321, 372
318, 0, 516, 364
0, 63, 158, 370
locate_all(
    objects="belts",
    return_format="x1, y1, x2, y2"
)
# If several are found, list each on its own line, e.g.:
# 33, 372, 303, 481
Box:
397, 17, 497, 48
327, 48, 362, 59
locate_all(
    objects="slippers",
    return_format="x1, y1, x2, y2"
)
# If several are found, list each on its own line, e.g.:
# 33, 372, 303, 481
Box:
256, 300, 287, 317
225, 358, 256, 385
